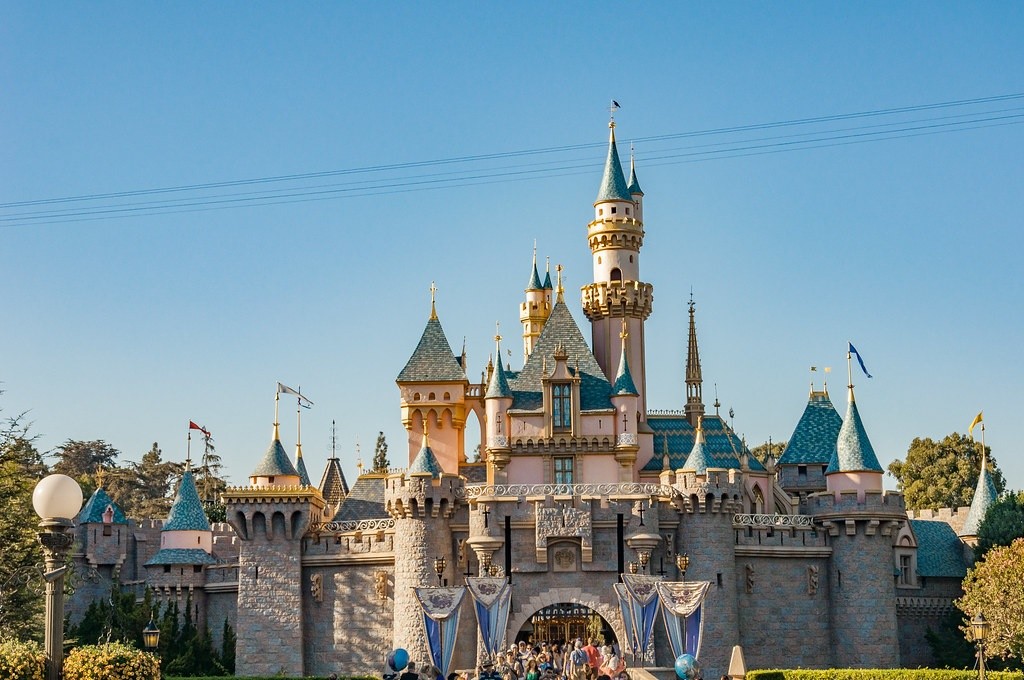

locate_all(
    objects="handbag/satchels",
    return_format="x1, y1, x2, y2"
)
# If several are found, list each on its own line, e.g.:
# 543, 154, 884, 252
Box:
583, 663, 591, 674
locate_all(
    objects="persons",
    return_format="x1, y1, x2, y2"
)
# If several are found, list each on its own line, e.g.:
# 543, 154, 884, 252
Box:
721, 675, 729, 680
383, 661, 465, 680
479, 637, 633, 680
673, 668, 703, 680
326, 673, 337, 680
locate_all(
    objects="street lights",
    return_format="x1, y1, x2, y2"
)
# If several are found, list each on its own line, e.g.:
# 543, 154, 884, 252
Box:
482, 553, 492, 659
32, 474, 84, 680
490, 565, 497, 661
675, 553, 690, 582
627, 560, 638, 668
970, 609, 989, 680
433, 556, 447, 672
638, 552, 651, 668
143, 610, 160, 655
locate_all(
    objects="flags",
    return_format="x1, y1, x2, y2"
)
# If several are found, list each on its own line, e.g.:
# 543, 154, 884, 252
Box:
278, 382, 315, 409
969, 410, 982, 435
190, 421, 211, 436
825, 367, 831, 373
849, 342, 873, 379
809, 366, 816, 371
612, 99, 622, 112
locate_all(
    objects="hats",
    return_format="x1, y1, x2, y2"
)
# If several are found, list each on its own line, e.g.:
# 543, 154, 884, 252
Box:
511, 644, 518, 649
525, 657, 539, 669
496, 652, 505, 658
542, 643, 546, 645
505, 650, 513, 655
482, 660, 494, 668
519, 641, 525, 645
